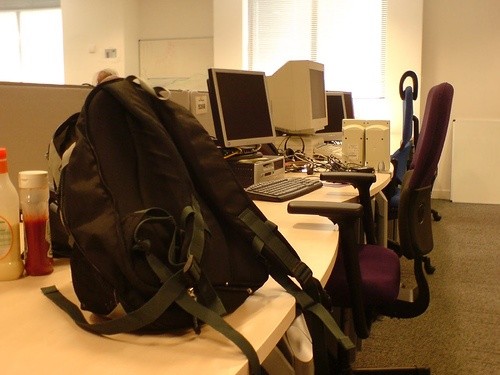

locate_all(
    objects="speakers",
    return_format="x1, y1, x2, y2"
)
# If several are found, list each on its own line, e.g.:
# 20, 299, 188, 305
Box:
341, 119, 390, 174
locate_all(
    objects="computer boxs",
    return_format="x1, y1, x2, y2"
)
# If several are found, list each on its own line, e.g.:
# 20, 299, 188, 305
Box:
228, 155, 284, 187
273, 137, 325, 156
314, 132, 343, 141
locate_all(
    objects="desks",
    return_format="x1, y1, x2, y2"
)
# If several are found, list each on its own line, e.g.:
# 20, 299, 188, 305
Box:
0, 144, 395, 375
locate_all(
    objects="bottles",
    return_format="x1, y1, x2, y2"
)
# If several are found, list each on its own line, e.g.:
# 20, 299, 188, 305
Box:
0, 147, 24, 281
18, 170, 54, 276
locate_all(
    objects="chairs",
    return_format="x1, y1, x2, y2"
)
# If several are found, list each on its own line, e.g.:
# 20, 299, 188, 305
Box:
287, 82, 454, 375
375, 71, 443, 222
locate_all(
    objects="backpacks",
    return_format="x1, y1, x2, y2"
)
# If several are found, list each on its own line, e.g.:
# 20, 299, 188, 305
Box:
39, 75, 354, 375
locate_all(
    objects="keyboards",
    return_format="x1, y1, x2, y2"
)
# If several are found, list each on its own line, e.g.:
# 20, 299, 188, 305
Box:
316, 144, 344, 157
245, 178, 323, 202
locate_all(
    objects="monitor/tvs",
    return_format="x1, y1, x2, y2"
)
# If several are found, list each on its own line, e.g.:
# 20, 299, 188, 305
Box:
315, 92, 348, 141
206, 67, 277, 161
325, 91, 354, 118
266, 60, 328, 138
